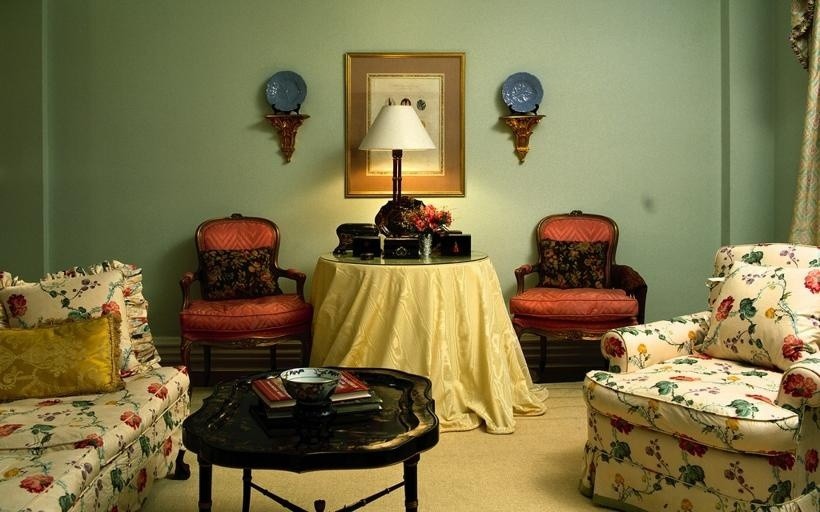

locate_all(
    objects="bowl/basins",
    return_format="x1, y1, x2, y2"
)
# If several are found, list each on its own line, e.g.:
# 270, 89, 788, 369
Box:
280, 367, 342, 406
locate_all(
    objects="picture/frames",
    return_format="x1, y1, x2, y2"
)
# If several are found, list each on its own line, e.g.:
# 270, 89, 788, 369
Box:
345, 51, 465, 198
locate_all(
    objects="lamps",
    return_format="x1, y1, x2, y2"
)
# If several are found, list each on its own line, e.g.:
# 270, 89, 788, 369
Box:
358, 105, 436, 237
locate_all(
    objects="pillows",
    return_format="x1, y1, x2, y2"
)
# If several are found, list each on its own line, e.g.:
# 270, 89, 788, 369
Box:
198, 245, 283, 301
0, 310, 127, 402
0, 259, 163, 378
700, 260, 820, 370
704, 243, 820, 310
536, 237, 609, 289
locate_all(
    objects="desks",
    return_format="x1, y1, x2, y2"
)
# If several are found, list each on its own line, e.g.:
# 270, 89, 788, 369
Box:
308, 250, 549, 435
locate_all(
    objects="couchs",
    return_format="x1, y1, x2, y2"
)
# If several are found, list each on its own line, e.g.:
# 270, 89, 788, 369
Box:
0, 364, 193, 511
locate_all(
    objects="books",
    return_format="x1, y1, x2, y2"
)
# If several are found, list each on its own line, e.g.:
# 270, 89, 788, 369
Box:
250, 370, 372, 410
265, 394, 385, 419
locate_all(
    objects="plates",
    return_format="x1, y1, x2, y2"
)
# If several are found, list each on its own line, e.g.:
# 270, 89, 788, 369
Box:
501, 72, 544, 113
264, 71, 308, 112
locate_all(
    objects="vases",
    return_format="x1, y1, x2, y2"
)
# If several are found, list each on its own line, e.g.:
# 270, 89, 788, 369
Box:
419, 232, 432, 257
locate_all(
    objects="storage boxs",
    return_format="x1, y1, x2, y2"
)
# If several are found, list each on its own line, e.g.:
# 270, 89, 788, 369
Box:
352, 235, 381, 257
434, 230, 462, 248
439, 233, 471, 256
384, 237, 420, 260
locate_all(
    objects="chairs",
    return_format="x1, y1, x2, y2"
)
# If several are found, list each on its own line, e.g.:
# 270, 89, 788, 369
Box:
179, 212, 314, 398
509, 209, 648, 379
578, 310, 820, 512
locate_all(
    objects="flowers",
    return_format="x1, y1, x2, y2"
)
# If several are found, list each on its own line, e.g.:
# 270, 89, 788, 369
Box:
395, 202, 453, 238
